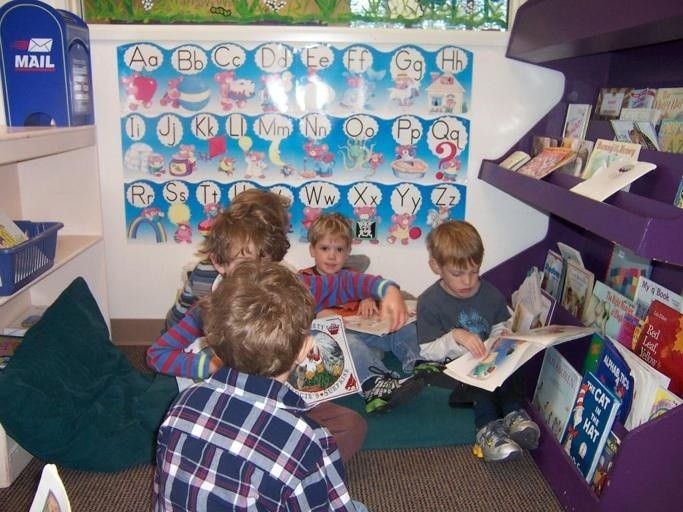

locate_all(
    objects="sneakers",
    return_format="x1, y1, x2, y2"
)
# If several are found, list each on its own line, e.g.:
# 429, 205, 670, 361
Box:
473, 419, 523, 464
503, 408, 541, 452
356, 365, 424, 416
412, 358, 462, 393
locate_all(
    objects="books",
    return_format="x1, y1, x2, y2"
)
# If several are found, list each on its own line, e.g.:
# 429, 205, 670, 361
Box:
174, 315, 363, 408
341, 300, 417, 338
541, 239, 683, 394
27, 464, 73, 512
531, 335, 683, 498
442, 321, 596, 394
500, 84, 683, 211
0, 207, 51, 287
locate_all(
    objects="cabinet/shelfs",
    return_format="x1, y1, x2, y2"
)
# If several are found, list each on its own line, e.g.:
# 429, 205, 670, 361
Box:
476, 1, 683, 512
0, 1, 116, 490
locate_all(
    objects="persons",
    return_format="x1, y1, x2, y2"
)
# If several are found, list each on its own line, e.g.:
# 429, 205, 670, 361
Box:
144, 202, 366, 465
151, 259, 366, 512
164, 188, 290, 338
295, 212, 442, 414
414, 219, 540, 464
